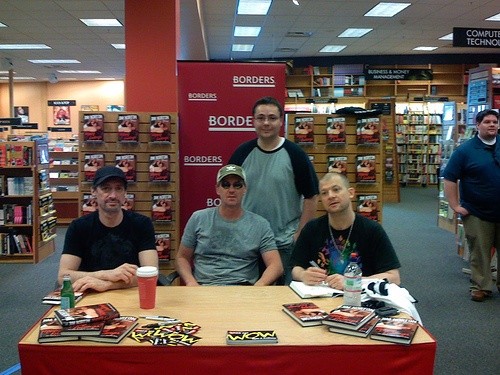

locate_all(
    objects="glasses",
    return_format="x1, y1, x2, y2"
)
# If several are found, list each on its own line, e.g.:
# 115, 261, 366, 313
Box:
220, 180, 244, 188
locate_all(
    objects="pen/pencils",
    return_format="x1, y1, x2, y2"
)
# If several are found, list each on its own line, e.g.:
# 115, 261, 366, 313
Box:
309, 260, 320, 269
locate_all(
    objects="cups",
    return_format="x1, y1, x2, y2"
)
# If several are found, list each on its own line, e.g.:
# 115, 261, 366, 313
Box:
137, 266, 159, 309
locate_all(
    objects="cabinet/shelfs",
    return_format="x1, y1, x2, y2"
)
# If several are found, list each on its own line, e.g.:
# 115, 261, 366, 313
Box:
394, 110, 467, 189
0, 140, 56, 264
47, 136, 80, 227
285, 63, 481, 105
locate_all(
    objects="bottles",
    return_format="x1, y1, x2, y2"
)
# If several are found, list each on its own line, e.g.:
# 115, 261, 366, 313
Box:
343, 256, 362, 306
60, 275, 75, 309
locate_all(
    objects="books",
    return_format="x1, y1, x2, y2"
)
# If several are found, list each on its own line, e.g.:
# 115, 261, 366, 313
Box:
36, 304, 136, 344
284, 58, 500, 287
282, 301, 420, 346
226, 330, 279, 346
1, 98, 175, 264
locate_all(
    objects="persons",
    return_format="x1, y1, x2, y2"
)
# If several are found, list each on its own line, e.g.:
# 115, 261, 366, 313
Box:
443, 109, 500, 301
289, 172, 403, 292
174, 164, 284, 286
58, 163, 160, 296
224, 97, 320, 288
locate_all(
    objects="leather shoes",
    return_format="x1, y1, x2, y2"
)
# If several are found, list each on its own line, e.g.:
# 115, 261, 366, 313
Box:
472, 290, 486, 301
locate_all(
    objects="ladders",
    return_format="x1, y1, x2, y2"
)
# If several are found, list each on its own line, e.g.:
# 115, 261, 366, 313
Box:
400, 88, 430, 188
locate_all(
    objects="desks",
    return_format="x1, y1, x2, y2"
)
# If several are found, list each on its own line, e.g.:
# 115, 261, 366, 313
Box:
17, 283, 438, 375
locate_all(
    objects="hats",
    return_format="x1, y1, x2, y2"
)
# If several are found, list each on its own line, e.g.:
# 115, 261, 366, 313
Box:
93, 166, 124, 187
217, 163, 246, 184
